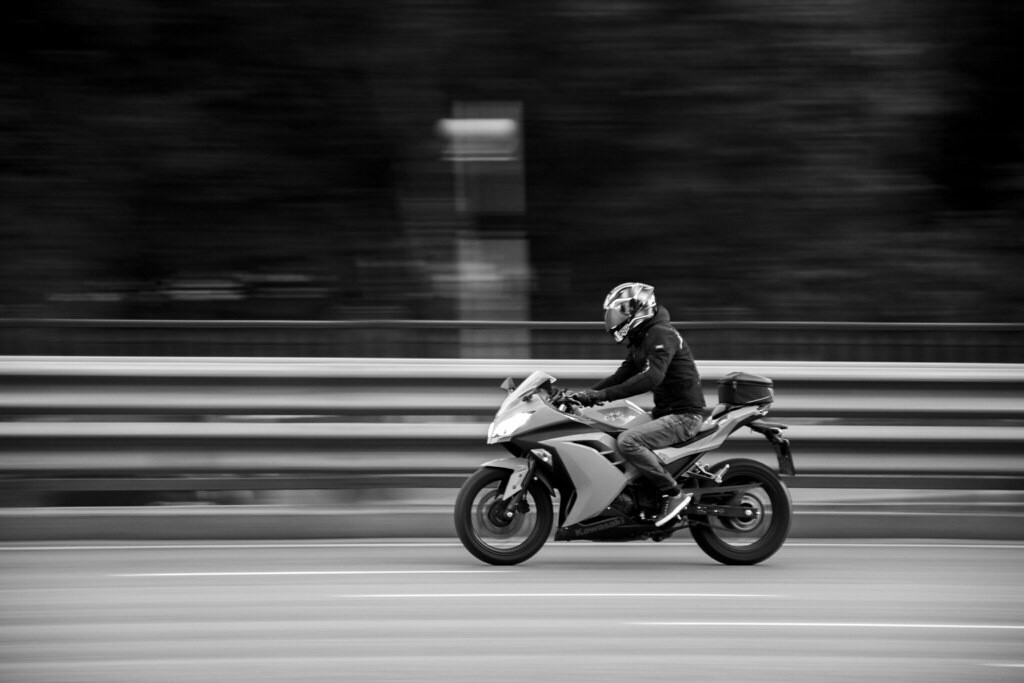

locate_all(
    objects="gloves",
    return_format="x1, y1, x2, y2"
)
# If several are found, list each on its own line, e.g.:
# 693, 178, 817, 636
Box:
574, 390, 600, 407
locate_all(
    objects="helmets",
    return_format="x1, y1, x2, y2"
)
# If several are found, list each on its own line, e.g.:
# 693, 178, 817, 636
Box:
603, 283, 656, 345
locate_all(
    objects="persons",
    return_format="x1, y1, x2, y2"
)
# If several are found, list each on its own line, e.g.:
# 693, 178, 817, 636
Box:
559, 281, 704, 526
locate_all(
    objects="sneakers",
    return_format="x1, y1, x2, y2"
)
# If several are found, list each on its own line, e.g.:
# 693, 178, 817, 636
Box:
655, 488, 691, 527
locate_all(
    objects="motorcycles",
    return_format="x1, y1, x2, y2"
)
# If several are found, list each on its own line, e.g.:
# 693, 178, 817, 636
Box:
452, 368, 796, 567
